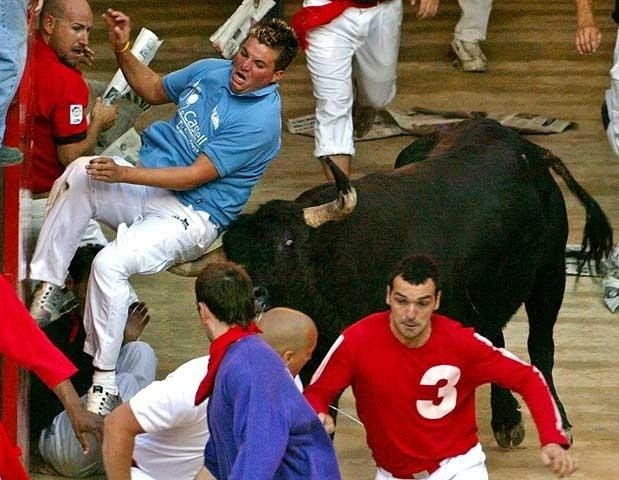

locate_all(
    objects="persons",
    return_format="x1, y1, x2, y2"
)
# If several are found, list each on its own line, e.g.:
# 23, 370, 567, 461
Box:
300, 0, 440, 183
0, 0, 156, 480
192, 261, 342, 480
303, 253, 579, 480
450, 0, 494, 71
574, 0, 619, 155
102, 308, 317, 480
28, 9, 298, 416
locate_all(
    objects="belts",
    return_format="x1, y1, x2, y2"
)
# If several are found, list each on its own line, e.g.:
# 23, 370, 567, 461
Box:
30, 191, 51, 199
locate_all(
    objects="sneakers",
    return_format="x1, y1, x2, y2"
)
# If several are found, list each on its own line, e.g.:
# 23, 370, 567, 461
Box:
29, 280, 81, 329
450, 37, 489, 72
85, 383, 123, 417
0, 143, 24, 168
350, 71, 377, 139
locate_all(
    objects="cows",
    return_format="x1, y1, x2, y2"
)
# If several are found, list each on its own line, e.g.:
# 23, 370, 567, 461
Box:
165, 116, 614, 449
